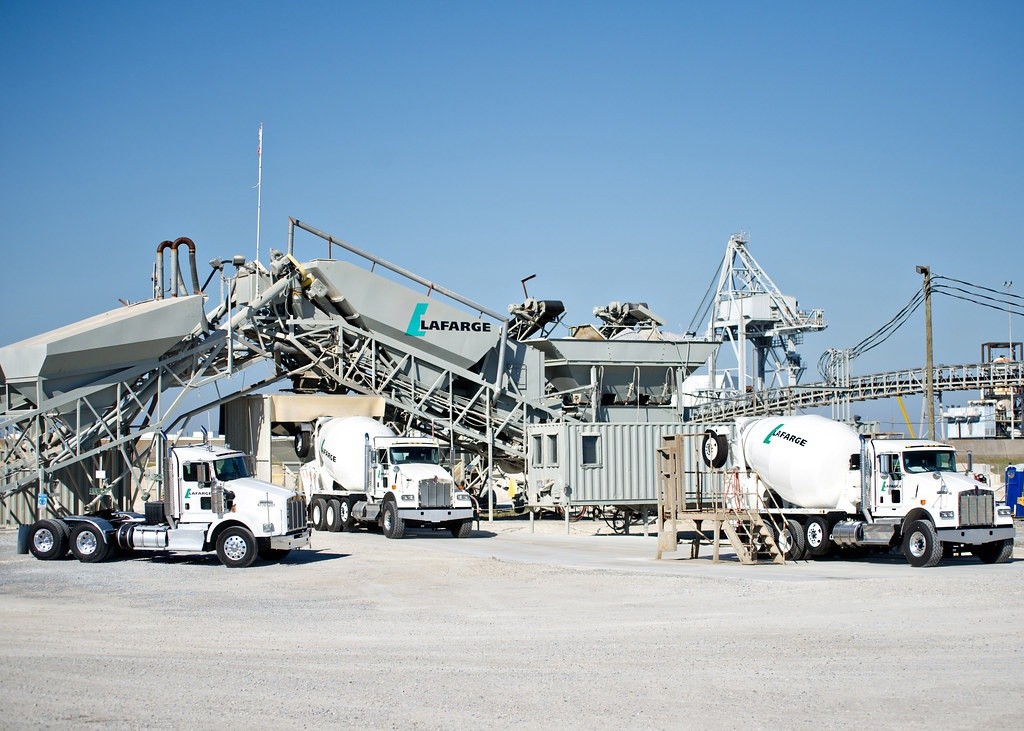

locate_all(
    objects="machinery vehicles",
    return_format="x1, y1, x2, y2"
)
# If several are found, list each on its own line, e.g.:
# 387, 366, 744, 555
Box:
701, 414, 1016, 568
17, 407, 313, 568
295, 415, 476, 539
